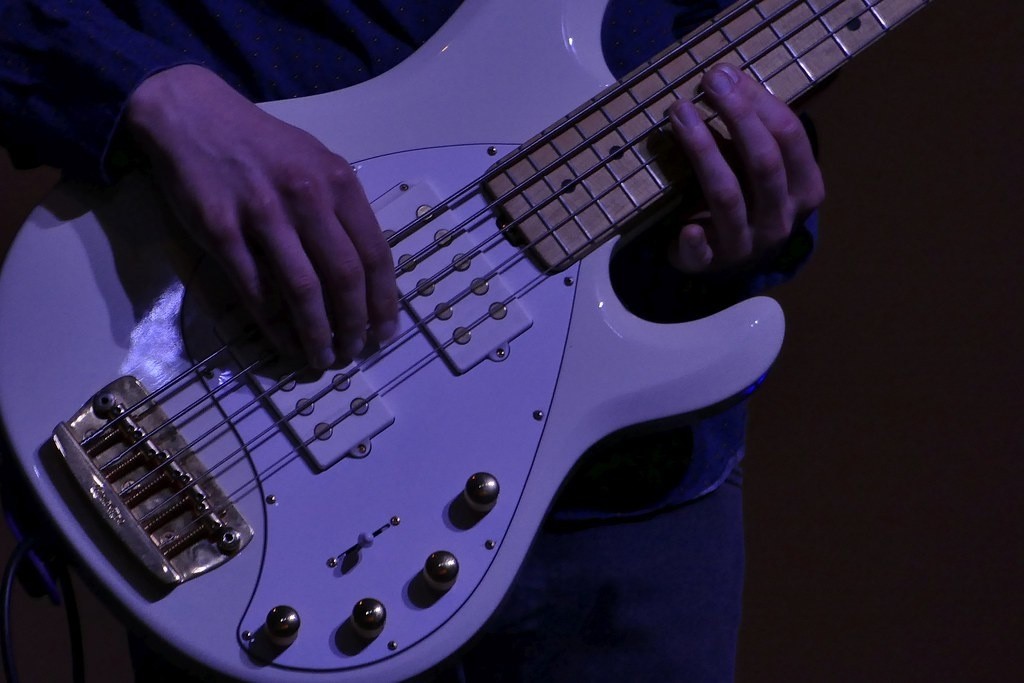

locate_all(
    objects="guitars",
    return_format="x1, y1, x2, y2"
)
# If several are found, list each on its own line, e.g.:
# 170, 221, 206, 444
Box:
0, 1, 932, 682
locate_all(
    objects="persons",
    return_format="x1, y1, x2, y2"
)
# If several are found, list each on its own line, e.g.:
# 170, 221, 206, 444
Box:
0, 0, 825, 683
